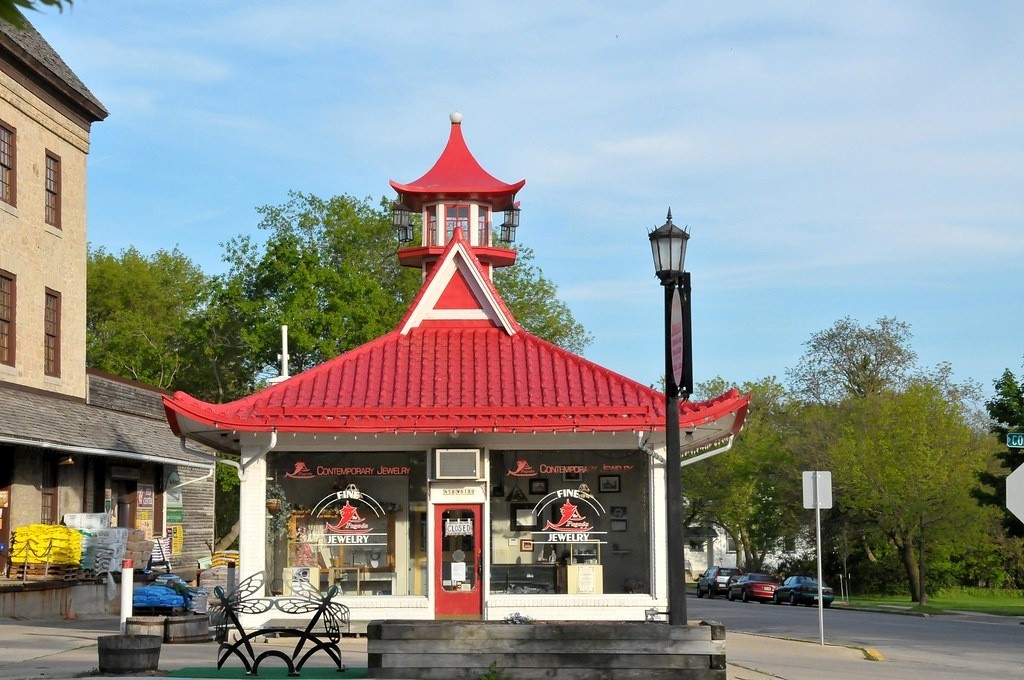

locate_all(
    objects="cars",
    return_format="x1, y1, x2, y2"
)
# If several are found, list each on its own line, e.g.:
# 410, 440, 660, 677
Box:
772, 574, 834, 609
727, 571, 780, 604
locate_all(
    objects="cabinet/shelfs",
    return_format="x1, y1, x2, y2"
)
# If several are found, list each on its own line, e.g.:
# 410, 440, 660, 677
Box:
289, 506, 399, 576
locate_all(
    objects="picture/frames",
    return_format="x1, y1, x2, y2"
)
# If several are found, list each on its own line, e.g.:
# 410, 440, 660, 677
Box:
529, 478, 549, 495
511, 504, 543, 532
563, 471, 583, 482
520, 539, 534, 552
598, 475, 621, 493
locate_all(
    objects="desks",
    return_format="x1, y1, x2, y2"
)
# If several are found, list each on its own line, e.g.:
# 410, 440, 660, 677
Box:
328, 565, 366, 594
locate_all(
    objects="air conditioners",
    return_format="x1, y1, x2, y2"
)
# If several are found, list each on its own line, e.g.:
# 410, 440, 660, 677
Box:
436, 449, 481, 480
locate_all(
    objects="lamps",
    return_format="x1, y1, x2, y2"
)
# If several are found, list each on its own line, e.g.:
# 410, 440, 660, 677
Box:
232, 438, 240, 449
685, 431, 693, 442
504, 451, 529, 503
500, 193, 521, 242
219, 433, 230, 444
392, 194, 414, 242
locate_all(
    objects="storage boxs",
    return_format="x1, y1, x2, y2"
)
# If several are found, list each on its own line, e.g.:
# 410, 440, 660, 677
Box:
63, 513, 155, 572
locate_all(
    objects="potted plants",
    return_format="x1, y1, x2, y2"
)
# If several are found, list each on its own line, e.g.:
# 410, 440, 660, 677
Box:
266, 484, 292, 545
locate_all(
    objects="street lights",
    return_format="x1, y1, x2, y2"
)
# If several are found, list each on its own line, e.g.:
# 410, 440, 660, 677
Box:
647, 205, 691, 627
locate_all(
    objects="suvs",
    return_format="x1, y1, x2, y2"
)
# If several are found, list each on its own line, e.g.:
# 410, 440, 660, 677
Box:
695, 564, 744, 599
